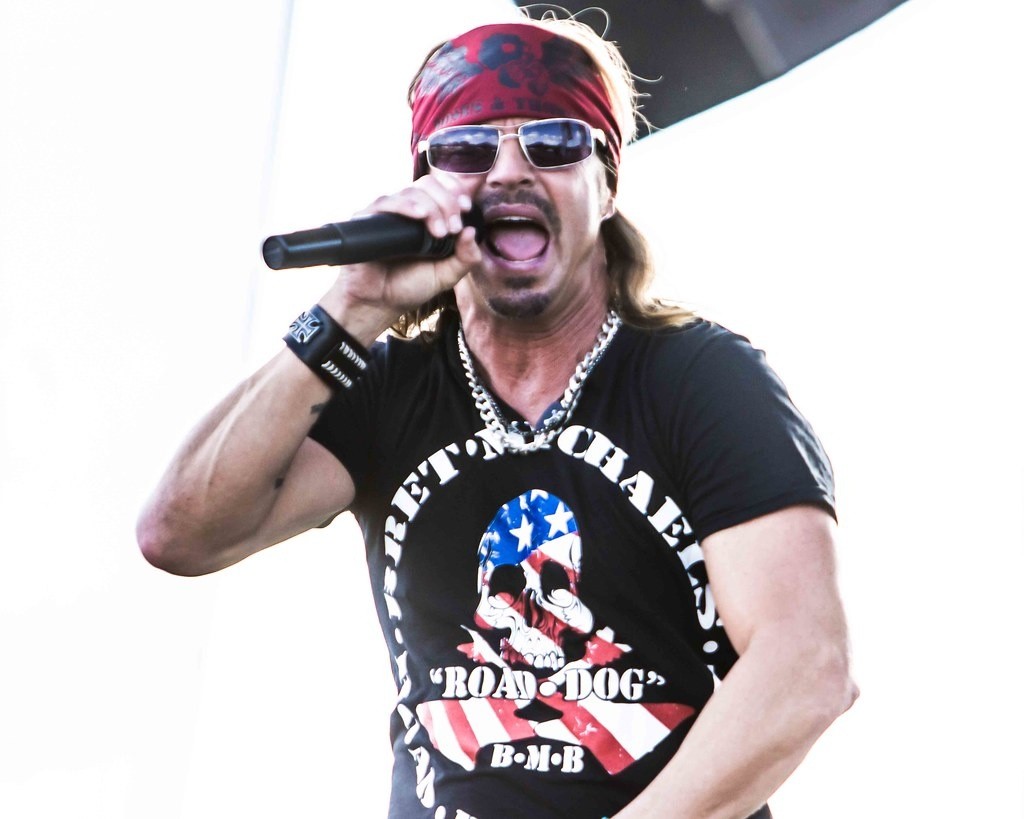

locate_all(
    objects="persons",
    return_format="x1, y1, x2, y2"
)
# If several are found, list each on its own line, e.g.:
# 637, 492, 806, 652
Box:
138, 18, 863, 819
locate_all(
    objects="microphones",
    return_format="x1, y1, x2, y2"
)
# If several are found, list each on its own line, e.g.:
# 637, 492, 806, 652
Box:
262, 203, 485, 270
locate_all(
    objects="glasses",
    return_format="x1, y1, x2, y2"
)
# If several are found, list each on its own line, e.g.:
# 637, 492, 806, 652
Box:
417, 118, 609, 175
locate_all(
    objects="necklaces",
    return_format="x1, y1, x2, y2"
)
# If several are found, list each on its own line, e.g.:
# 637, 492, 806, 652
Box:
440, 312, 617, 453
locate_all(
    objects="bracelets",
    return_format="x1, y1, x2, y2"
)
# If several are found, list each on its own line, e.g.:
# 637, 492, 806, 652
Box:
280, 305, 372, 393
